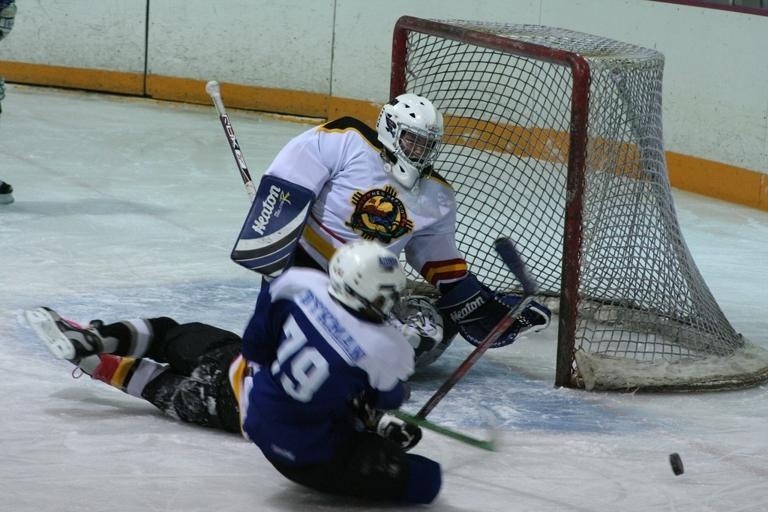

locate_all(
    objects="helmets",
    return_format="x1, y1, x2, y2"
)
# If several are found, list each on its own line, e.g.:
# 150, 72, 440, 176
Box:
375, 93, 444, 153
327, 239, 407, 321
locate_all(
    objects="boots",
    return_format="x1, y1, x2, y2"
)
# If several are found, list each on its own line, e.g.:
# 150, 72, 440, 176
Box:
35, 306, 103, 366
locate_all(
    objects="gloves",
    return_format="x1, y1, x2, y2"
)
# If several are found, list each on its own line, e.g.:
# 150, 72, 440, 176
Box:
431, 271, 552, 348
365, 408, 422, 451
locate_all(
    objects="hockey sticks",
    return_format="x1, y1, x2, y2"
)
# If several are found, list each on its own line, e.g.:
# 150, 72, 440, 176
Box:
414, 236, 539, 429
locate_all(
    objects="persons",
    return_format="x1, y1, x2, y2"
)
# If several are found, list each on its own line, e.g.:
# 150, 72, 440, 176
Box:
1, 0, 16, 195
224, 93, 552, 375
26, 293, 445, 438
233, 239, 446, 508
